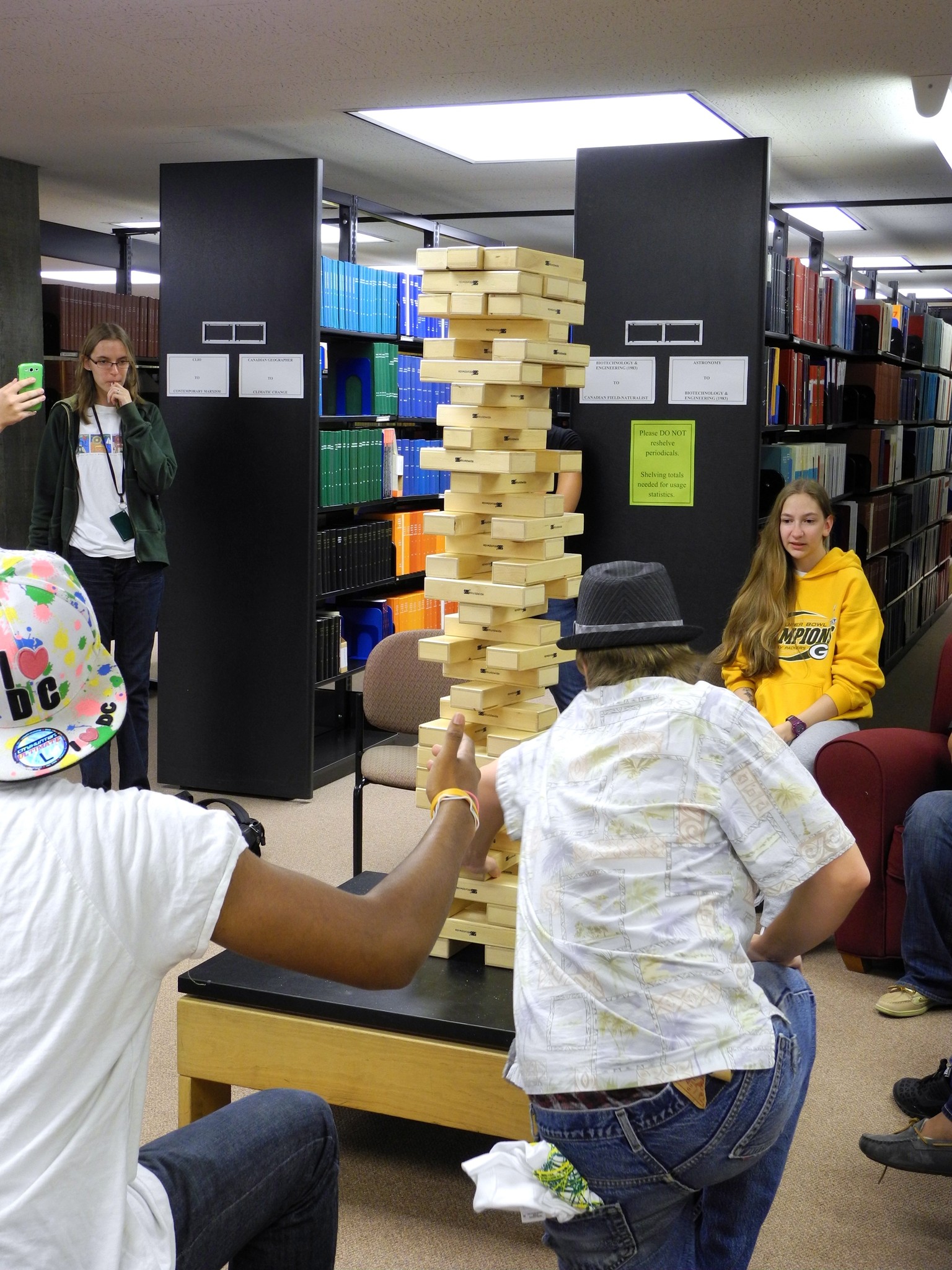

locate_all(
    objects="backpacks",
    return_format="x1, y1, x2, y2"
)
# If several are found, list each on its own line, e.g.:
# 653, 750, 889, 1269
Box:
177, 789, 265, 857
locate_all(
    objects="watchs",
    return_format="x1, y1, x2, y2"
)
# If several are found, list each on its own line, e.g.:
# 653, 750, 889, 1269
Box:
785, 715, 807, 740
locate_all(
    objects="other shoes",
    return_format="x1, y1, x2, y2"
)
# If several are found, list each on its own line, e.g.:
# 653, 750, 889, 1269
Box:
859, 1117, 952, 1178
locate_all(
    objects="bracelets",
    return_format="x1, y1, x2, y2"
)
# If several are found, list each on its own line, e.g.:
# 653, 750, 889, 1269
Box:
430, 788, 479, 831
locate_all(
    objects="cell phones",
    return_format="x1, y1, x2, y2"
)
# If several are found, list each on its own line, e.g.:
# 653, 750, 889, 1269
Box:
17, 363, 43, 412
110, 510, 135, 542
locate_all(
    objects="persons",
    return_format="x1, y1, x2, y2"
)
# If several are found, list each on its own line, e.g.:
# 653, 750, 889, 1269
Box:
713, 479, 885, 774
0, 548, 481, 1269
463, 561, 870, 1270
875, 733, 952, 1017
27, 323, 178, 789
859, 1055, 952, 1178
547, 425, 588, 714
0, 377, 46, 433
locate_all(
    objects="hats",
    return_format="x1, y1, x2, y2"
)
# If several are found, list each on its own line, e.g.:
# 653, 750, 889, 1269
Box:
556, 560, 702, 651
1, 546, 129, 779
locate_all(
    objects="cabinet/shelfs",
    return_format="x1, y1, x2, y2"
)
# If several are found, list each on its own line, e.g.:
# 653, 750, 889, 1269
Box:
150, 150, 498, 805
561, 133, 952, 678
0, 156, 160, 554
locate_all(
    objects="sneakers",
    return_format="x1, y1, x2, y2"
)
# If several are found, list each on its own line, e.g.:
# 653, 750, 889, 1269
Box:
875, 985, 938, 1016
891, 1058, 952, 1119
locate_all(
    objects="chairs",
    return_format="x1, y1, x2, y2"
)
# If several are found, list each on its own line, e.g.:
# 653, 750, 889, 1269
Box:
350, 626, 473, 877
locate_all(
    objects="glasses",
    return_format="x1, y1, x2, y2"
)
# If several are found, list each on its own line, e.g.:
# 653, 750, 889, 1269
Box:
87, 356, 133, 369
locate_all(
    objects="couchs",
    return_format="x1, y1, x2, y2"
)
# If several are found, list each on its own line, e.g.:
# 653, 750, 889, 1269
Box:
809, 632, 952, 973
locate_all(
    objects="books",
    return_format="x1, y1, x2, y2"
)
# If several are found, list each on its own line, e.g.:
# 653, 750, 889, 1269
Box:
765, 251, 952, 661
319, 342, 451, 417
42, 284, 159, 358
44, 360, 81, 422
316, 589, 458, 682
318, 510, 445, 597
320, 422, 451, 506
321, 256, 449, 338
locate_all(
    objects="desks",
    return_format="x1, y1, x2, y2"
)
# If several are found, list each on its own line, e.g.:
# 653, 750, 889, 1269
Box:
168, 868, 537, 1147
